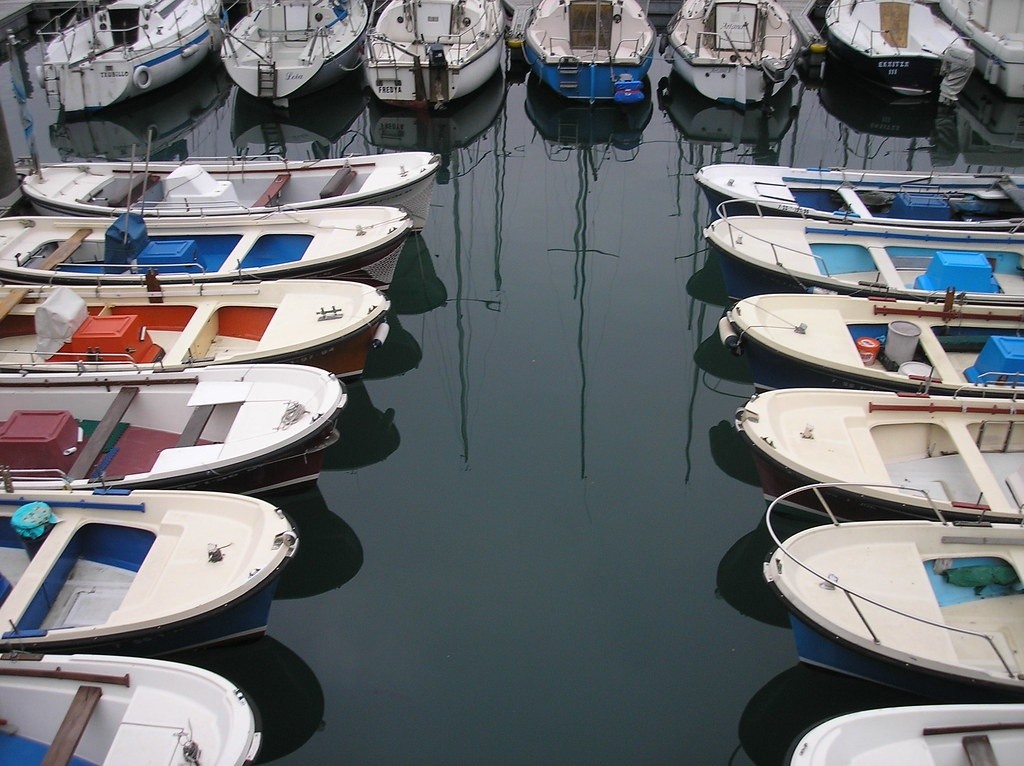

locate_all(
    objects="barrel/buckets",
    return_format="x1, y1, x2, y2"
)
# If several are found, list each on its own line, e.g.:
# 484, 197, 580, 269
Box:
885, 320, 921, 366
856, 337, 880, 365
898, 361, 935, 378
10, 503, 55, 563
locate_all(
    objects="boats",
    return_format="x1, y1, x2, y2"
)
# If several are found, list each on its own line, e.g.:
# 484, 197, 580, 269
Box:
17, 148, 442, 233
781, 703, 1024, 766
827, 2, 974, 105
735, 384, 1024, 524
704, 198, 1024, 303
367, 0, 513, 109
663, 4, 803, 111
0, 489, 302, 651
0, 652, 264, 765
215, 0, 367, 103
0, 204, 413, 289
0, 364, 347, 496
54, 70, 1021, 480
696, 164, 1024, 229
940, 0, 1024, 100
762, 521, 1023, 694
716, 291, 1024, 397
42, 0, 224, 118
517, 0, 657, 107
0, 278, 392, 379
